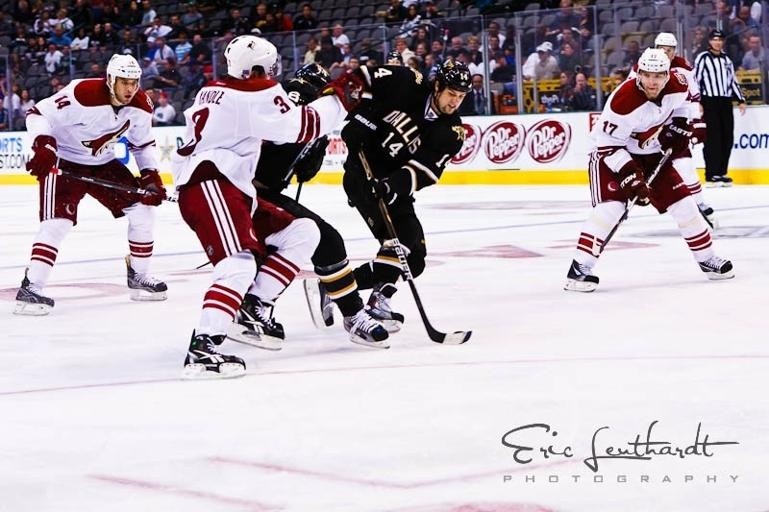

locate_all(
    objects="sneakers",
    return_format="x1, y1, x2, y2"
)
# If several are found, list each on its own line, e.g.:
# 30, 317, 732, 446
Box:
699, 202, 714, 215
567, 259, 598, 283
698, 256, 732, 273
705, 174, 732, 186
342, 307, 389, 341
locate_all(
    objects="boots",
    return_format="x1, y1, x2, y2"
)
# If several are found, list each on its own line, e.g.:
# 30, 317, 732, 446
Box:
234, 282, 284, 338
124, 255, 167, 293
303, 276, 334, 327
364, 283, 404, 324
16, 267, 54, 306
184, 329, 245, 373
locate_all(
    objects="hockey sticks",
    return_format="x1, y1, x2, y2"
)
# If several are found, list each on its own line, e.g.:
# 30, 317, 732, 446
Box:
355, 151, 471, 344
592, 148, 672, 256
194, 137, 319, 271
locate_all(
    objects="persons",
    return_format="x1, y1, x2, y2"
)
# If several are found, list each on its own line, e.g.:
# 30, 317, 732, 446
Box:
170, 34, 366, 382
691, 28, 747, 189
12, 53, 169, 316
2, 0, 768, 133
302, 58, 473, 334
562, 47, 736, 293
625, 32, 715, 222
250, 61, 391, 351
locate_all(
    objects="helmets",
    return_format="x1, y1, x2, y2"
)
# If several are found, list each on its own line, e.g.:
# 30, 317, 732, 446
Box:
635, 47, 670, 98
289, 62, 329, 93
107, 54, 141, 107
437, 59, 473, 92
655, 31, 677, 49
225, 36, 281, 80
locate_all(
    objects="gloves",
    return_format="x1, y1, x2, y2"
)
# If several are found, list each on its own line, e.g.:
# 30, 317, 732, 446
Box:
660, 120, 694, 155
365, 169, 412, 206
26, 136, 57, 181
619, 162, 652, 205
293, 136, 330, 183
135, 171, 166, 205
341, 114, 376, 162
321, 72, 364, 110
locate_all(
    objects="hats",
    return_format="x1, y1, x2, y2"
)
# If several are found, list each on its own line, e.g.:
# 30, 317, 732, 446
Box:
710, 30, 725, 37
